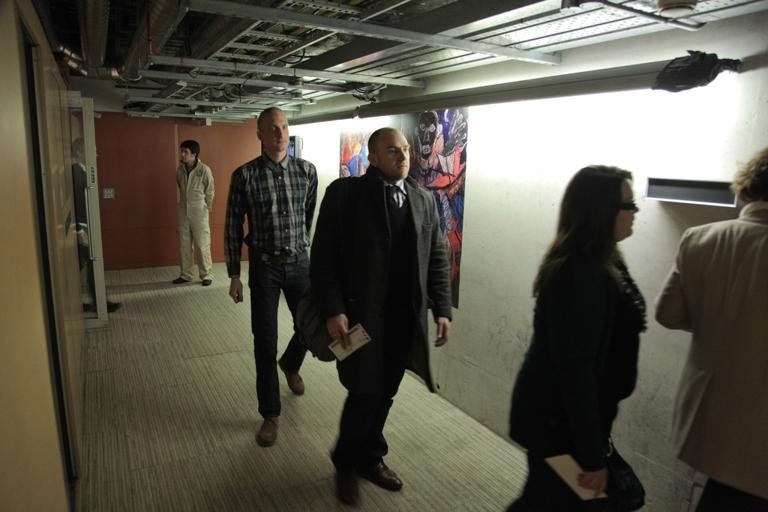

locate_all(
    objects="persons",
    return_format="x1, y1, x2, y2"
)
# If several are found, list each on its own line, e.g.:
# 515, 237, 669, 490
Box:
307, 126, 453, 511
652, 149, 765, 510
173, 139, 216, 287
224, 107, 318, 446
500, 165, 651, 511
68, 137, 123, 314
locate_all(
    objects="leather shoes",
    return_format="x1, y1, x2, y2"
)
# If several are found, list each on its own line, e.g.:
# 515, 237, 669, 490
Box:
173, 277, 187, 284
202, 279, 212, 285
364, 461, 402, 491
105, 300, 119, 311
334, 475, 358, 504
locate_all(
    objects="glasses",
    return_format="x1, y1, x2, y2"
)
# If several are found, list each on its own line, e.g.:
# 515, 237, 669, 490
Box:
619, 201, 635, 210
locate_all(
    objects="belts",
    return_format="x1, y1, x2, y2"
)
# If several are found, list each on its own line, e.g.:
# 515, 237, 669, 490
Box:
270, 253, 307, 264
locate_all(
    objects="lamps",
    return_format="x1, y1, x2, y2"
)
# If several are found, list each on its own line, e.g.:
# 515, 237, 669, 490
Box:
286, 105, 360, 126
358, 49, 740, 119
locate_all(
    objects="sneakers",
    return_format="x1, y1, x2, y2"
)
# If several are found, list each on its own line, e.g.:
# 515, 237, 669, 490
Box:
284, 371, 304, 394
255, 417, 278, 445
